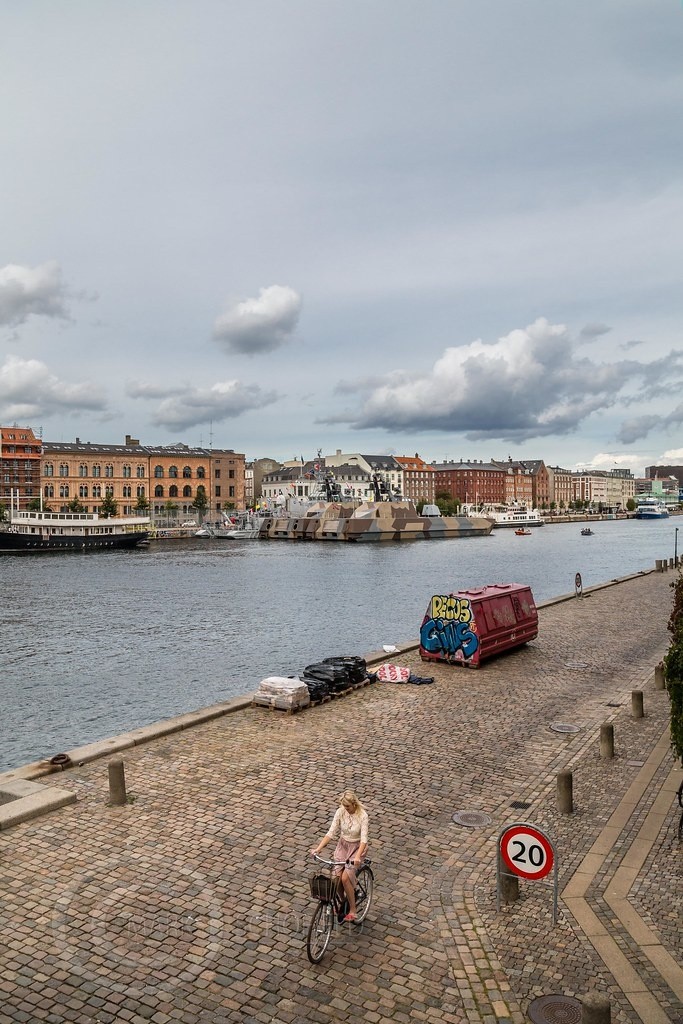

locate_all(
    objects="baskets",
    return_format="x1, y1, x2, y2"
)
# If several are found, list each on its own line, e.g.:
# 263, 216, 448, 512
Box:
308, 871, 340, 901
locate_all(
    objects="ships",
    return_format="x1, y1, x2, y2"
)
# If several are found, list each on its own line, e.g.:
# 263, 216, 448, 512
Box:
466, 500, 544, 527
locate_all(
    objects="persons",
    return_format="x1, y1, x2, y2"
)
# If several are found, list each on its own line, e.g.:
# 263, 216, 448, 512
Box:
215, 517, 220, 529
312, 790, 369, 921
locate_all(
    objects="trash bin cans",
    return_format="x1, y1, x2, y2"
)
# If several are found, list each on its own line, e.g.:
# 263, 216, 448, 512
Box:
419, 582, 539, 669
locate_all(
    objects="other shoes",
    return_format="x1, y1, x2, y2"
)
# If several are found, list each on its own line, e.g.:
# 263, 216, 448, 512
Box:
344, 913, 358, 922
333, 903, 341, 915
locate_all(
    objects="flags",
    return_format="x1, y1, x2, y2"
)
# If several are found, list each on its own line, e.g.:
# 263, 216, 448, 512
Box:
248, 464, 320, 513
330, 470, 373, 501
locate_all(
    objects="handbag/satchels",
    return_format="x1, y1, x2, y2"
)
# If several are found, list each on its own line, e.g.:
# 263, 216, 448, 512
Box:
313, 875, 335, 898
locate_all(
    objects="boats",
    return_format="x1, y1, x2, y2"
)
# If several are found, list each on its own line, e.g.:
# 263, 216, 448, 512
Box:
0, 486, 151, 552
581, 527, 594, 535
195, 513, 258, 539
315, 467, 495, 542
258, 470, 363, 540
514, 529, 531, 536
635, 495, 670, 519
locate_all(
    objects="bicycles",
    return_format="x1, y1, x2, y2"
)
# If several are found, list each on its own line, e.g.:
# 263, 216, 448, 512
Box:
307, 851, 374, 963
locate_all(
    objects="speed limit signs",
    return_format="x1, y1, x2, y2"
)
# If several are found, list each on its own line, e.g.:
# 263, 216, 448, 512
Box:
502, 826, 553, 881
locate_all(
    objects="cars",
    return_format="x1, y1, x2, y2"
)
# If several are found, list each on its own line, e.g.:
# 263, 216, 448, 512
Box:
159, 519, 223, 529
